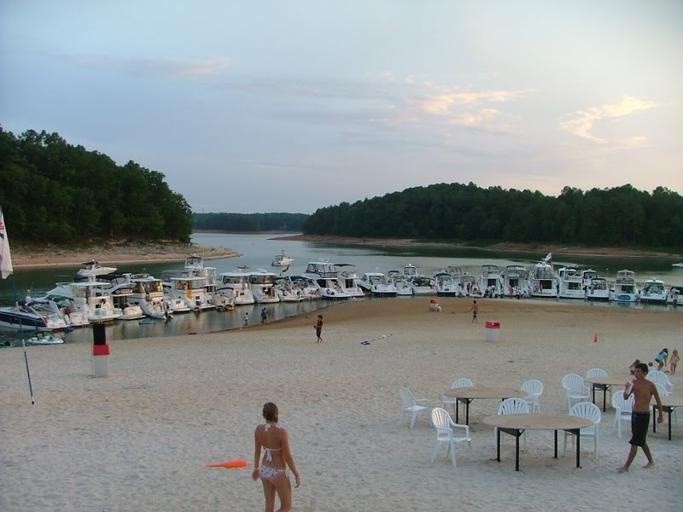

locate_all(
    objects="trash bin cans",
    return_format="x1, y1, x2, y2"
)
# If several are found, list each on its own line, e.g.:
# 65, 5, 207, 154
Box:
430, 300, 439, 311
485, 321, 501, 341
92, 344, 111, 377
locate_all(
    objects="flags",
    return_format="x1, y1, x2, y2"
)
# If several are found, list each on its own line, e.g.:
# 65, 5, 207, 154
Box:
0, 210, 13, 279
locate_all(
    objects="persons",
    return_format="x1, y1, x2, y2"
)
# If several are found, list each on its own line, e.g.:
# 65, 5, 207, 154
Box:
625, 348, 680, 379
252, 402, 301, 512
467, 300, 479, 324
617, 364, 663, 473
313, 315, 324, 342
241, 307, 271, 327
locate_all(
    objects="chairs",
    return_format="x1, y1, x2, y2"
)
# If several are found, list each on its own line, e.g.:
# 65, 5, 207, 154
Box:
398, 386, 432, 430
494, 367, 673, 462
431, 407, 473, 469
440, 377, 473, 422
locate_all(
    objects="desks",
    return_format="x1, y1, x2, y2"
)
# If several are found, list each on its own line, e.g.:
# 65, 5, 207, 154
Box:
443, 385, 528, 425
649, 396, 683, 441
483, 413, 593, 471
